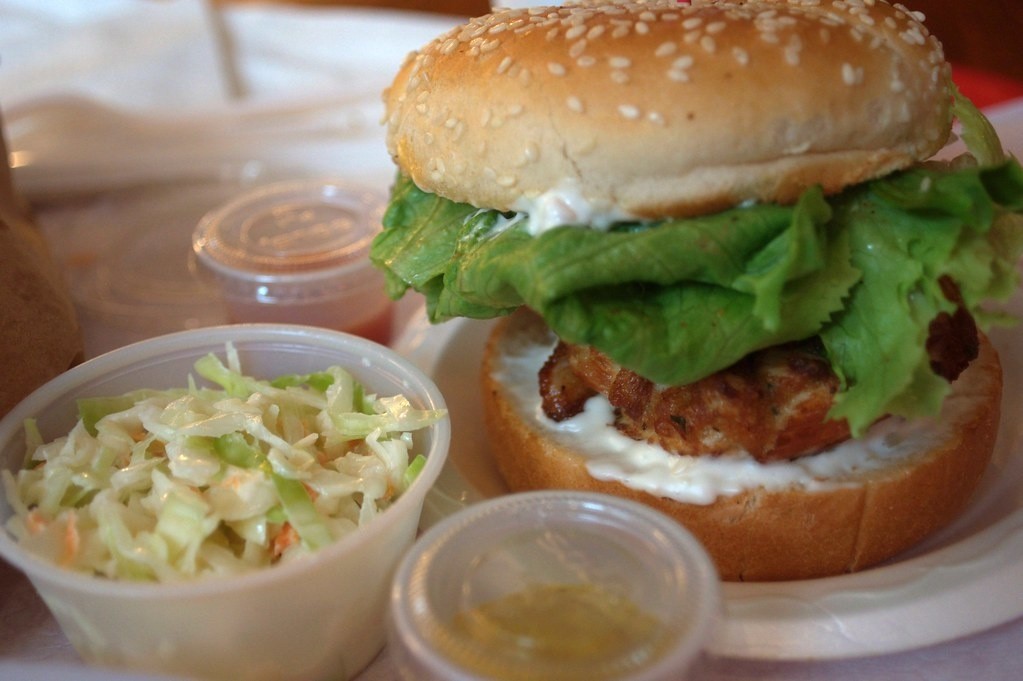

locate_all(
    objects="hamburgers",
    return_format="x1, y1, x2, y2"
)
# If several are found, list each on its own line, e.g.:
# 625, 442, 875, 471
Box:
369, 0, 1023, 584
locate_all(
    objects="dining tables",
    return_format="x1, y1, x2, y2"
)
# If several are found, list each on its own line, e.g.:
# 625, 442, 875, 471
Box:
0, 0, 1022, 677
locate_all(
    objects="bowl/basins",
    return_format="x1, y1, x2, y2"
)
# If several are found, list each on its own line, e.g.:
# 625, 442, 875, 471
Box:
389, 490, 721, 681
188, 180, 393, 344
2, 319, 453, 681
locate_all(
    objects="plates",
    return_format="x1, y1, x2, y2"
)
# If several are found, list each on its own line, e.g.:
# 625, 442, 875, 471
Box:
394, 282, 1022, 661
